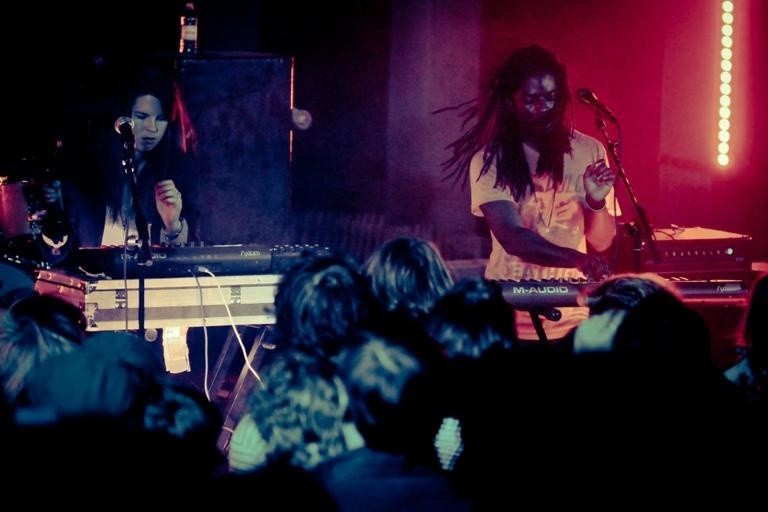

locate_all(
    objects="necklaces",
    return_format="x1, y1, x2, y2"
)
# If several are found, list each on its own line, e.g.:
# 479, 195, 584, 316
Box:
533, 183, 557, 234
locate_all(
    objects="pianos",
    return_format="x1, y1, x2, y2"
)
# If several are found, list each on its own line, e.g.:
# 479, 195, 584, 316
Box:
491, 276, 747, 310
63, 242, 330, 282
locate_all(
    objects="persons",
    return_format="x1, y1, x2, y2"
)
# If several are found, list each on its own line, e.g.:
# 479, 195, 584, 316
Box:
432, 43, 623, 342
44, 83, 203, 379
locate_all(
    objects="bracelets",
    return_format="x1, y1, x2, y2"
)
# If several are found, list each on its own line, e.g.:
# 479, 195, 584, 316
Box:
165, 230, 182, 238
586, 193, 606, 211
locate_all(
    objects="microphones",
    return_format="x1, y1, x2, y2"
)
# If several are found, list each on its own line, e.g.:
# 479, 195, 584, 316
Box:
575, 84, 620, 130
112, 112, 139, 152
